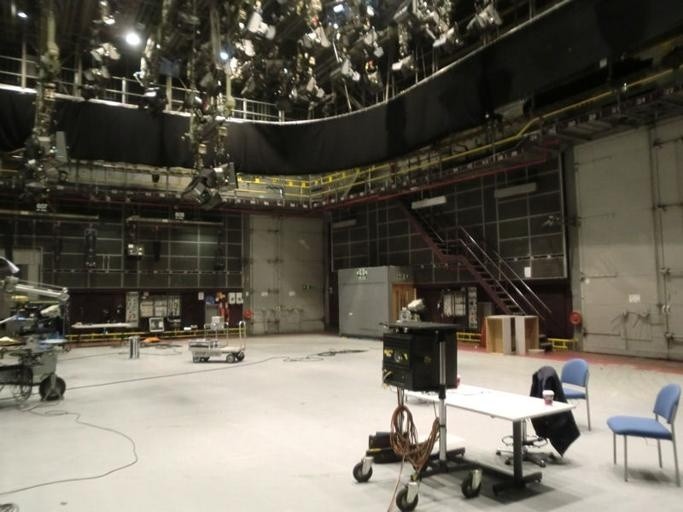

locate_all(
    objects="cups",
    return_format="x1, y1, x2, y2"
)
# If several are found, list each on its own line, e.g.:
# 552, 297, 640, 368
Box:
541, 390, 555, 408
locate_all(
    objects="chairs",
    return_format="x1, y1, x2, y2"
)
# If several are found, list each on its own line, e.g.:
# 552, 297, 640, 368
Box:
494, 363, 562, 467
605, 381, 682, 491
560, 357, 591, 430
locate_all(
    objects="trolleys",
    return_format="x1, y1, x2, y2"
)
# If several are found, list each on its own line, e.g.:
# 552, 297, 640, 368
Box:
187, 319, 246, 363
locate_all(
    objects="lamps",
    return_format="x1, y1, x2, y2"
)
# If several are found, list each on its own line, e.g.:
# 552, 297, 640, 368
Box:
239, 0, 503, 114
6, 0, 239, 219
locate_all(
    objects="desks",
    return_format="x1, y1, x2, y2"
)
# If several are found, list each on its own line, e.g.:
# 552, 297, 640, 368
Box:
388, 381, 577, 496
